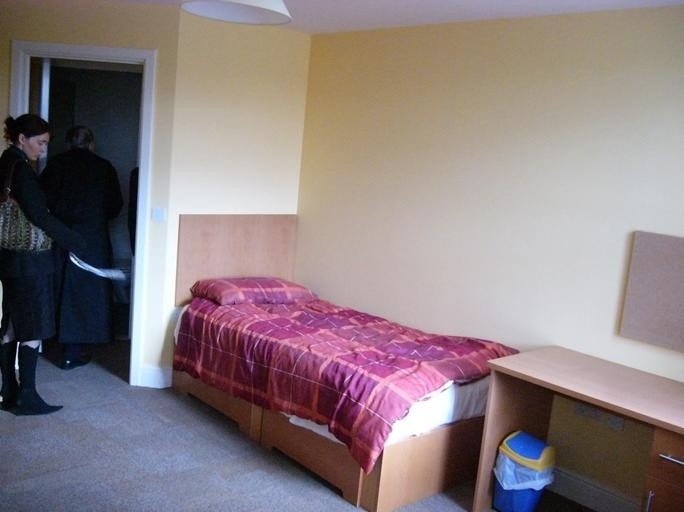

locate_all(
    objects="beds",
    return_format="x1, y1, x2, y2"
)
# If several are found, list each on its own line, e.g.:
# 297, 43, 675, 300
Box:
170, 297, 520, 512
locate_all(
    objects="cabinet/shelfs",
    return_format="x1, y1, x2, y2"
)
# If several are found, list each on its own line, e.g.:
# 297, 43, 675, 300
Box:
637, 425, 683, 512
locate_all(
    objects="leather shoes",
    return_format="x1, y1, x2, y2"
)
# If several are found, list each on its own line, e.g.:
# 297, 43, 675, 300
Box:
61, 354, 92, 369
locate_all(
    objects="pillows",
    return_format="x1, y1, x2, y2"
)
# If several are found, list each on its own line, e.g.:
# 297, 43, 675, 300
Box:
188, 277, 320, 306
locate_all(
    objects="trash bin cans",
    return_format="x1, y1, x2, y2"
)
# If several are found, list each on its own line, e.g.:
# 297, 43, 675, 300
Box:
490, 429, 557, 511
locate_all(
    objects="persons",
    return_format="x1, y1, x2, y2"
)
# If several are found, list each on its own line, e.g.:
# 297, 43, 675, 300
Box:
128, 166, 139, 256
39, 126, 125, 366
0, 112, 88, 417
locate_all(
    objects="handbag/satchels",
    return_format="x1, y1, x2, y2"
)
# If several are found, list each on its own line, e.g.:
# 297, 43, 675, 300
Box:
0, 197, 53, 253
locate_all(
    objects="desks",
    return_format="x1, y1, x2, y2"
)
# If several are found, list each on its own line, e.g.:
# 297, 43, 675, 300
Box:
470, 344, 683, 512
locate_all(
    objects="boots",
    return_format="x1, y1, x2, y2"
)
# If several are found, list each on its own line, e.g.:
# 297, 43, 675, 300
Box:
0, 341, 63, 416
